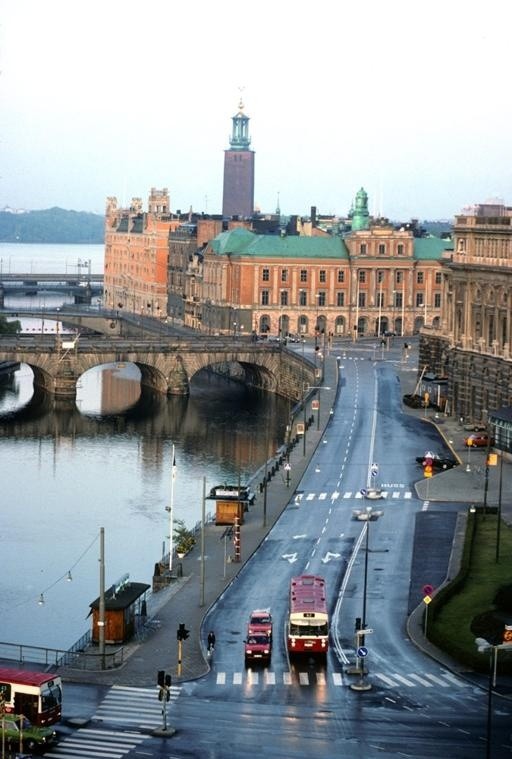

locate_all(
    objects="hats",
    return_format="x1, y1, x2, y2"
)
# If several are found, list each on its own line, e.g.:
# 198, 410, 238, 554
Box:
415, 451, 459, 471
462, 432, 493, 448
264, 335, 309, 346
242, 632, 275, 662
244, 609, 275, 636
381, 331, 396, 337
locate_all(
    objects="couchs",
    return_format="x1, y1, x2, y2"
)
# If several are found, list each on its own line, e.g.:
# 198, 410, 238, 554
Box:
180, 623, 191, 641
157, 668, 172, 689
362, 623, 369, 629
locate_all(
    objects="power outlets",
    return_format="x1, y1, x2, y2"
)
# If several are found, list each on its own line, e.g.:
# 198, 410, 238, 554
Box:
463, 422, 487, 432
0, 707, 55, 752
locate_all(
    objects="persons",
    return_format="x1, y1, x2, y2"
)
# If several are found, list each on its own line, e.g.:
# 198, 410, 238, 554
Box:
207, 631, 215, 656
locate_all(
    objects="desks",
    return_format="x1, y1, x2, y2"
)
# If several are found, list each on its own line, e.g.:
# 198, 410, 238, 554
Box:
473, 637, 512, 759
419, 303, 428, 327
232, 319, 237, 344
158, 308, 164, 336
165, 319, 168, 337
140, 305, 144, 324
351, 506, 384, 688
55, 304, 61, 349
301, 383, 335, 460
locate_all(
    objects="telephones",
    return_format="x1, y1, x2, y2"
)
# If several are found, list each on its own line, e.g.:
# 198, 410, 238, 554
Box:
357, 629, 374, 635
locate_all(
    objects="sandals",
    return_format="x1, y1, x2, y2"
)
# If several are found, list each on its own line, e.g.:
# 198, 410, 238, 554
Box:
0, 666, 65, 723
287, 575, 331, 656
